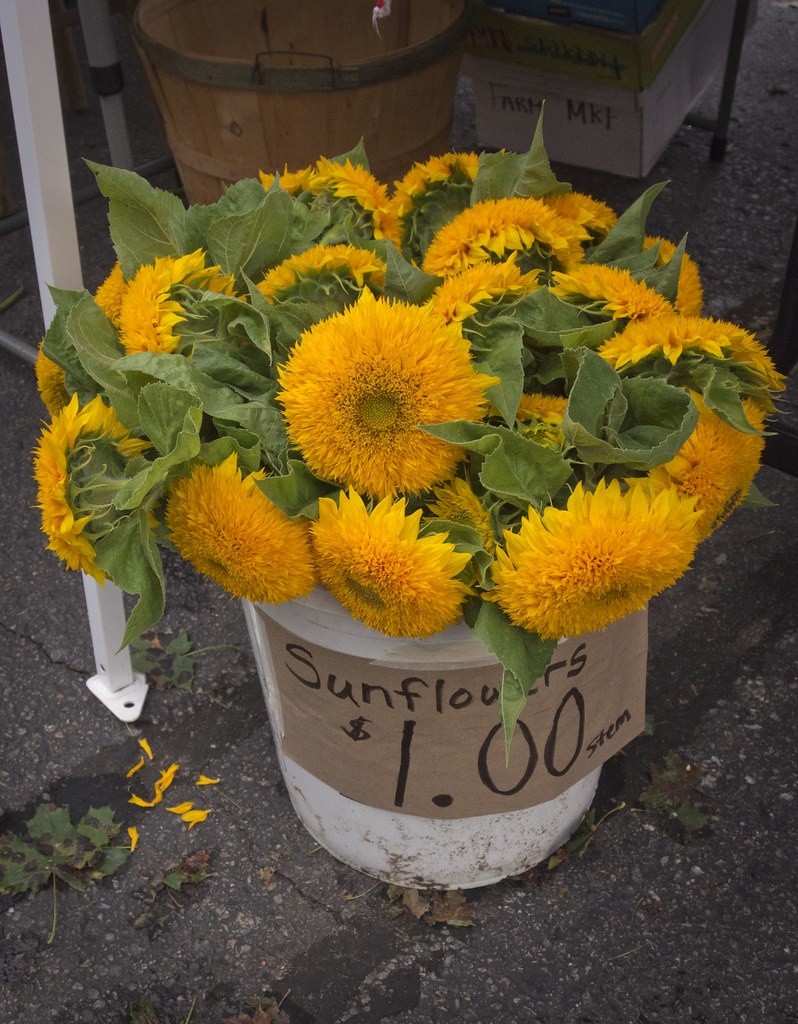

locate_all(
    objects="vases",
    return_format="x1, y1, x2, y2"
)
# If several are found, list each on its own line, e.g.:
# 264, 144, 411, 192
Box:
240, 592, 650, 893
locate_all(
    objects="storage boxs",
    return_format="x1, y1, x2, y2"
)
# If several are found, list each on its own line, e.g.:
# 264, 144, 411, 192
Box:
475, 0, 703, 95
475, 0, 737, 177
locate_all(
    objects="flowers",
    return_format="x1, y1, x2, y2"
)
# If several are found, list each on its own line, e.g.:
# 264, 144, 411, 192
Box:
31, 102, 790, 769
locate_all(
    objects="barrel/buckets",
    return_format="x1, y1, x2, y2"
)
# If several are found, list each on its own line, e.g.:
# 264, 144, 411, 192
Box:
133, 0, 470, 206
